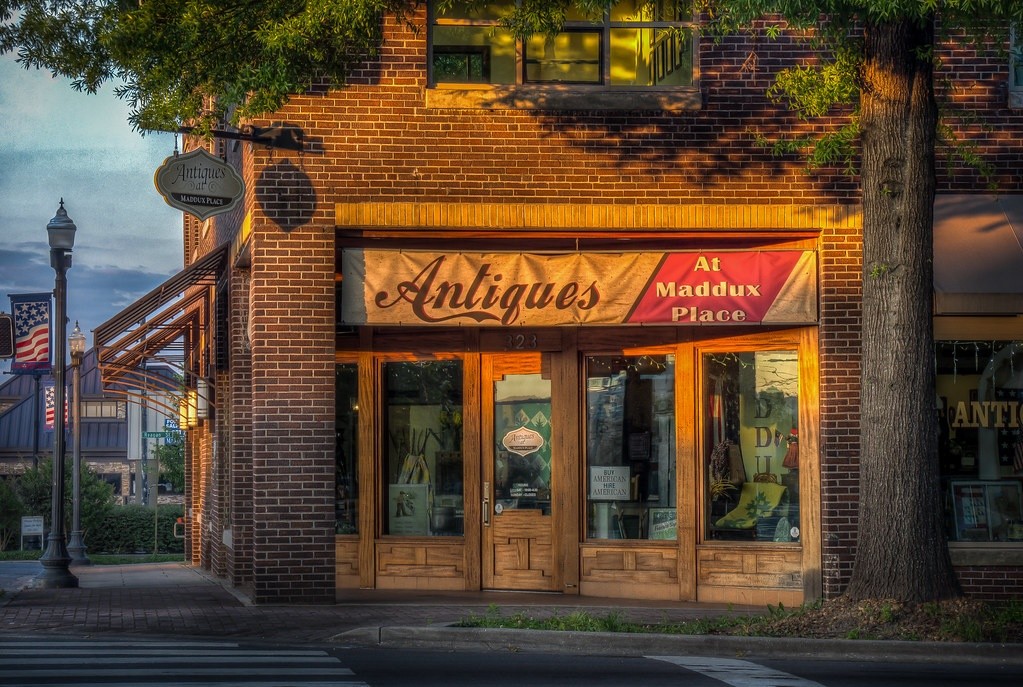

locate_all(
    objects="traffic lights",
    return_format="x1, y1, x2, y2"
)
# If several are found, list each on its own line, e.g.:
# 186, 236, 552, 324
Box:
0, 314, 15, 359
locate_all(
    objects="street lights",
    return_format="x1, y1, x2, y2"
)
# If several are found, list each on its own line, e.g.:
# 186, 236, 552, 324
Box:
64, 319, 91, 565
28, 197, 81, 588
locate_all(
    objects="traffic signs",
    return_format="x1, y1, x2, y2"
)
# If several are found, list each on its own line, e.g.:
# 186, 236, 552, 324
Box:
142, 432, 172, 438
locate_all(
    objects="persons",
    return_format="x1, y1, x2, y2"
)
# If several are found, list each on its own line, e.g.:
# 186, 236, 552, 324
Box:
395, 491, 407, 518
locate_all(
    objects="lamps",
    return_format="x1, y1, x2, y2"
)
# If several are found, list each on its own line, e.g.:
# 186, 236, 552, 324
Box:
180, 377, 210, 430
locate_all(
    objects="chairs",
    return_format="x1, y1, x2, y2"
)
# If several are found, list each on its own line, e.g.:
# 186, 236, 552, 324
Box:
710, 481, 791, 543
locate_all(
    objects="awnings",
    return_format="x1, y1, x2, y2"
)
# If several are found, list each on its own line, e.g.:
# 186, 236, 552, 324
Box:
98, 281, 211, 410
102, 308, 200, 427
93, 240, 231, 411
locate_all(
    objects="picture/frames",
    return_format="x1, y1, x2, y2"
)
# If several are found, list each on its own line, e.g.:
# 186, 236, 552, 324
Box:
647, 506, 678, 540
388, 484, 431, 536
586, 464, 633, 503
946, 477, 1023, 543
